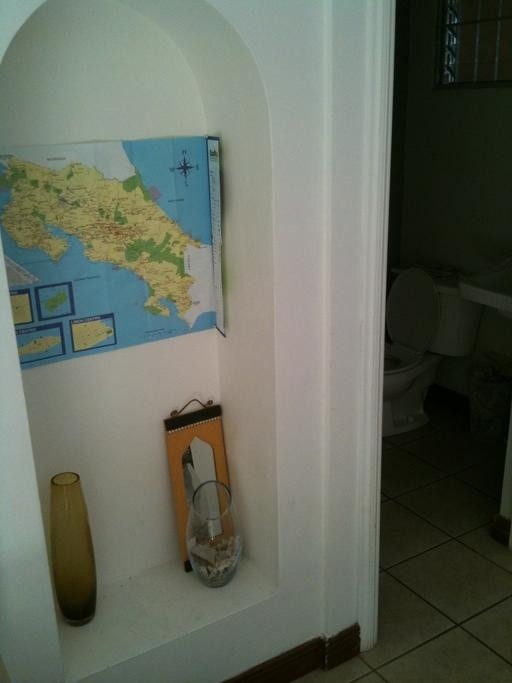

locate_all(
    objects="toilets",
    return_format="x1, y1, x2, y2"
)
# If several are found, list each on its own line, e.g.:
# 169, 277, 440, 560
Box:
383, 267, 483, 438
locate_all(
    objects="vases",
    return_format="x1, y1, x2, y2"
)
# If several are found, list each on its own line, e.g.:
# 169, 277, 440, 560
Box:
187, 480, 243, 588
51, 472, 97, 628
